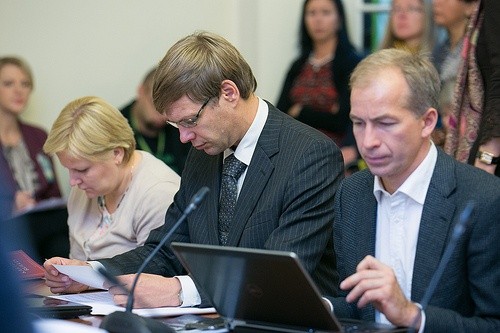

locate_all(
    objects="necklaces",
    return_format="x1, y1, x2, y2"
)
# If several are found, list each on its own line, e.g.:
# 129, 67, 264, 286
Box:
309, 50, 333, 71
116, 154, 135, 207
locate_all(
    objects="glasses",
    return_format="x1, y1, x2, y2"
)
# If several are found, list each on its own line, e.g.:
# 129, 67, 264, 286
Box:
166, 96, 211, 129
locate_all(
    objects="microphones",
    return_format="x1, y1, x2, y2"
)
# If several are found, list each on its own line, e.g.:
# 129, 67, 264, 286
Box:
100, 187, 212, 333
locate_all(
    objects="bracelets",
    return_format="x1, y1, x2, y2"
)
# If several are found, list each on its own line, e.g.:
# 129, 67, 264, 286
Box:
409, 309, 421, 333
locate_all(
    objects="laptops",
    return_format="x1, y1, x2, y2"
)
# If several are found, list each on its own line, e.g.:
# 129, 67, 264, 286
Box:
170, 241, 417, 333
26, 296, 92, 320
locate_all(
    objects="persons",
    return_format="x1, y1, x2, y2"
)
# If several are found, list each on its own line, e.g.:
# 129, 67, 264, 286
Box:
0, 0, 500, 333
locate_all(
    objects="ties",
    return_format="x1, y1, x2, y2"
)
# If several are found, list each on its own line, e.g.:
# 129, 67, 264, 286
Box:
218, 153, 248, 246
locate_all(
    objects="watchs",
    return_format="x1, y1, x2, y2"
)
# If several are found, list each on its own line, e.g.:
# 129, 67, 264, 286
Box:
175, 276, 183, 306
476, 150, 500, 166
